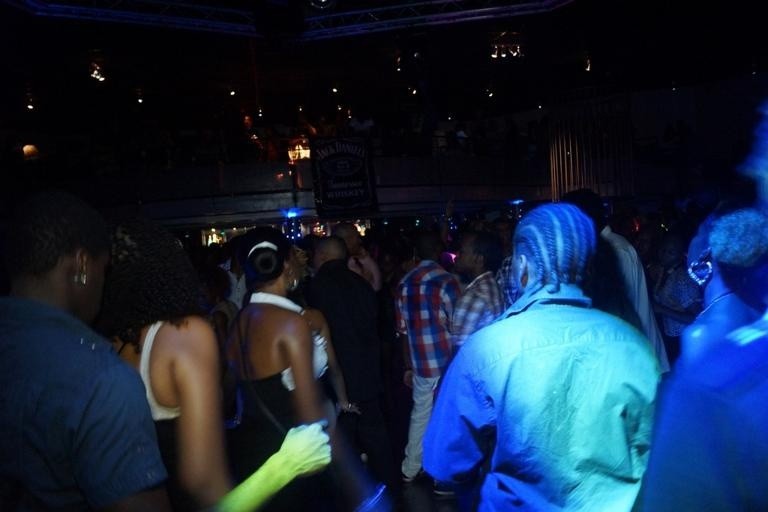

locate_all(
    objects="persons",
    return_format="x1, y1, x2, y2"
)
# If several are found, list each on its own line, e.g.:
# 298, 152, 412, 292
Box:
1, 187, 767, 510
